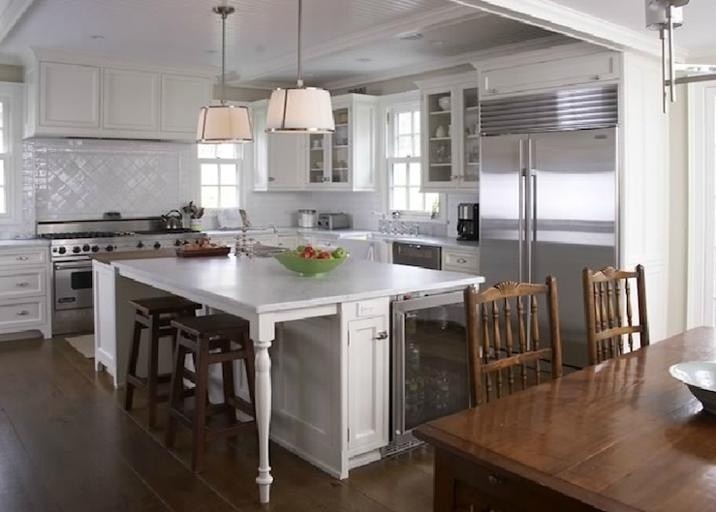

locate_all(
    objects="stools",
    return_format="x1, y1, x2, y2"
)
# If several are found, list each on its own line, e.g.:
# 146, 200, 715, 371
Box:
123, 296, 202, 431
166, 313, 262, 474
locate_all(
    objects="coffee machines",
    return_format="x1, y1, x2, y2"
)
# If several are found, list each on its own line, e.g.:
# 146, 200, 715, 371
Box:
456, 202, 479, 242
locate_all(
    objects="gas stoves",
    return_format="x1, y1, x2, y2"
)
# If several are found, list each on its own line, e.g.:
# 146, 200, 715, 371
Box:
37, 217, 208, 262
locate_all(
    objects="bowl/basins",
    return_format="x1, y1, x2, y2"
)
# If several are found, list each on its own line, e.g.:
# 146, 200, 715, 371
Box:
666, 359, 715, 416
315, 161, 325, 168
438, 96, 451, 111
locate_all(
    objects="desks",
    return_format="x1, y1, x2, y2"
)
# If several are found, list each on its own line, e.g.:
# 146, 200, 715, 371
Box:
91, 252, 487, 505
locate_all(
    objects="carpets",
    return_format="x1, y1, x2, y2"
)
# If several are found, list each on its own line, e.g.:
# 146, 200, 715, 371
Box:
64, 334, 95, 359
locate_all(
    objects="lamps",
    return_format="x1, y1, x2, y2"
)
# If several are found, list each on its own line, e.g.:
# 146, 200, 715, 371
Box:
263, 0, 337, 135
195, 0, 256, 143
645, 0, 692, 113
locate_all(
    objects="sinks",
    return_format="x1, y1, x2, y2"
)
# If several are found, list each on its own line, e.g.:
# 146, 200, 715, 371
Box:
341, 230, 408, 241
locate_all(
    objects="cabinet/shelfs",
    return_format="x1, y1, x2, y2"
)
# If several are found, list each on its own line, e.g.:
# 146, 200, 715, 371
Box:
413, 71, 479, 195
441, 243, 480, 328
209, 232, 391, 263
251, 98, 303, 191
23, 60, 216, 141
0, 237, 53, 343
305, 92, 376, 191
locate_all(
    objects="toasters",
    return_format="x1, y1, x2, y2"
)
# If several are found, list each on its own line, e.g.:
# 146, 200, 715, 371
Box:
317, 212, 350, 231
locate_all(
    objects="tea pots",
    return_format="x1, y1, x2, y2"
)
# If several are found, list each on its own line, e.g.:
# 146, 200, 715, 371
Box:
160, 209, 183, 232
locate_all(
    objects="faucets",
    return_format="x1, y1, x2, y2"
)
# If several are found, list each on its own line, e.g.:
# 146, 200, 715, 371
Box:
389, 210, 401, 233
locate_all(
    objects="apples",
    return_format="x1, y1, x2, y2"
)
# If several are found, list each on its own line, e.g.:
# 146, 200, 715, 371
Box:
333, 248, 346, 258
300, 246, 330, 260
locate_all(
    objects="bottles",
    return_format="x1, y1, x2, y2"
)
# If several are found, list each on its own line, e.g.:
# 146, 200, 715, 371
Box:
436, 124, 445, 137
447, 124, 452, 137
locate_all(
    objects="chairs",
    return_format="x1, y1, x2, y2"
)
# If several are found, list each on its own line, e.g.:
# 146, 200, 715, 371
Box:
465, 276, 563, 409
583, 264, 649, 366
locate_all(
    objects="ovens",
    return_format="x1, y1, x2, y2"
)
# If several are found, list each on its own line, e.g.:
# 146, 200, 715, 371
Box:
50, 260, 94, 339
